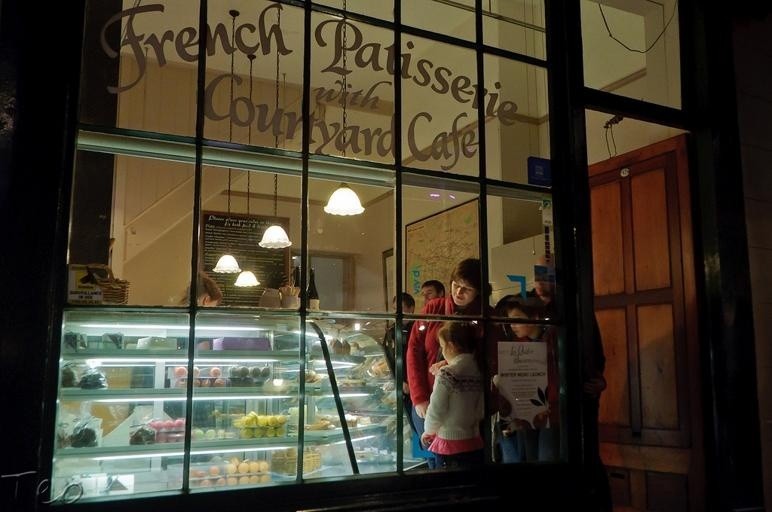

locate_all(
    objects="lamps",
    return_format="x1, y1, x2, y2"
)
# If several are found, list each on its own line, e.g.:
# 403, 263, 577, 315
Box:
254, 2, 288, 251
234, 46, 260, 290
325, 0, 365, 220
213, 13, 245, 275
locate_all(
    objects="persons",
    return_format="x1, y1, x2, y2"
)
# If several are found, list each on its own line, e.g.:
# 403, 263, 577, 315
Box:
143, 269, 225, 428
504, 295, 562, 469
381, 291, 417, 436
406, 257, 501, 447
492, 251, 607, 463
421, 320, 490, 468
400, 279, 448, 418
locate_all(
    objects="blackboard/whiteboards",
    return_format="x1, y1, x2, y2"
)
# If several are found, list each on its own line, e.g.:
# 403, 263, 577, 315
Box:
200, 210, 291, 307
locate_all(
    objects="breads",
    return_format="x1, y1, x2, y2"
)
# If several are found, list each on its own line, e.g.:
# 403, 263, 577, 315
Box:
227, 457, 269, 486
305, 340, 365, 430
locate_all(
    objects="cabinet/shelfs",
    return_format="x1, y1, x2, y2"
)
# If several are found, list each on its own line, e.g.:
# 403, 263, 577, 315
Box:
51, 304, 429, 502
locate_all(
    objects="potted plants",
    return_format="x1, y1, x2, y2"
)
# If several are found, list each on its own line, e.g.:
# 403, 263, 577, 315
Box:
259, 263, 283, 308
